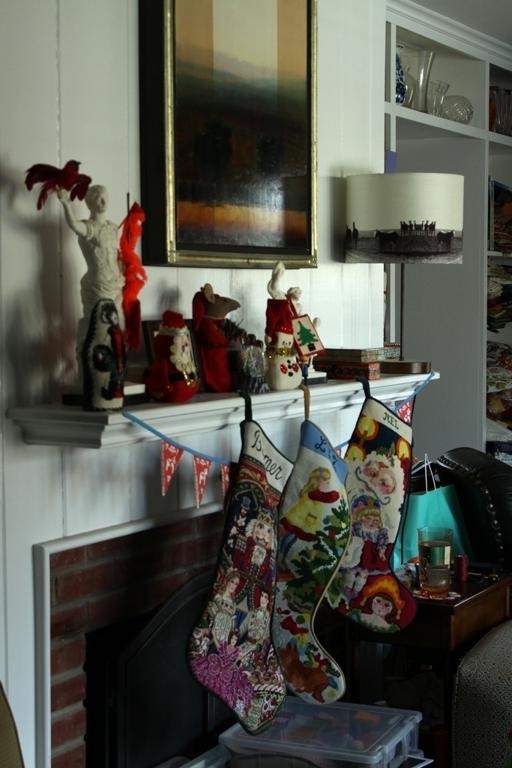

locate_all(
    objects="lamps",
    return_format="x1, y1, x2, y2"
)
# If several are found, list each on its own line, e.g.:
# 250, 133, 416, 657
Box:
346, 172, 465, 373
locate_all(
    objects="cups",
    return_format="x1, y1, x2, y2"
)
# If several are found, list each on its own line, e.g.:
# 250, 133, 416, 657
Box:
418, 528, 452, 596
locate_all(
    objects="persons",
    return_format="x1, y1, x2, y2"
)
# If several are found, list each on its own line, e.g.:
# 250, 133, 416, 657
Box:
399, 219, 436, 237
211, 569, 242, 646
55, 183, 125, 355
233, 590, 271, 656
278, 466, 342, 582
350, 577, 402, 636
350, 494, 389, 573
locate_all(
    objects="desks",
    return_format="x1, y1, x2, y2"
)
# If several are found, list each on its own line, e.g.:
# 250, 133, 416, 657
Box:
345, 571, 512, 768
181, 744, 425, 768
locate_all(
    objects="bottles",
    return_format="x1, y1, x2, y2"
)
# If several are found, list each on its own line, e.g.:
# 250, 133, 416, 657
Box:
456, 553, 467, 582
488, 85, 512, 136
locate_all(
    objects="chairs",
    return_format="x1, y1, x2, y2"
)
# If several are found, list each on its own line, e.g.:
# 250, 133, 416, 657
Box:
436, 447, 512, 572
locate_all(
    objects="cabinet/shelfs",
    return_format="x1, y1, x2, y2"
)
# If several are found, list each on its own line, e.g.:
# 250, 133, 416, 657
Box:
384, 0, 512, 467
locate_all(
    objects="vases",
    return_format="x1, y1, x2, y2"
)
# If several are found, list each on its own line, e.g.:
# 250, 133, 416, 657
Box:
396, 44, 406, 106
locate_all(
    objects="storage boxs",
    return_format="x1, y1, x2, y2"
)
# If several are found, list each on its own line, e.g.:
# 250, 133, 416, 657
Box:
218, 695, 422, 768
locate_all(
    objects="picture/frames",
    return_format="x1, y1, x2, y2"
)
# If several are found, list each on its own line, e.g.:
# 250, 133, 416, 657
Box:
139, 0, 317, 269
